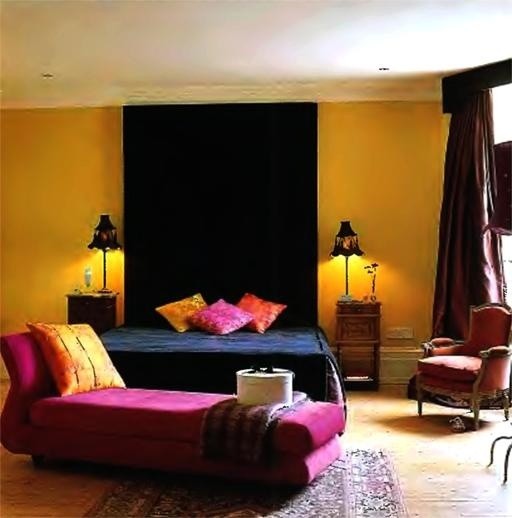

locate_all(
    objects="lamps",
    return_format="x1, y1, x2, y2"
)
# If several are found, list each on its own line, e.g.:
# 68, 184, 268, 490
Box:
88, 213, 123, 295
328, 218, 364, 305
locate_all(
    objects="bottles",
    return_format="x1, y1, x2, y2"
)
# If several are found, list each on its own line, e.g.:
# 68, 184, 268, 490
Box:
85, 268, 91, 288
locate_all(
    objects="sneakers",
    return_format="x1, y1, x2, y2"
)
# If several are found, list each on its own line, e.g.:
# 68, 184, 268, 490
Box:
449, 416, 466, 433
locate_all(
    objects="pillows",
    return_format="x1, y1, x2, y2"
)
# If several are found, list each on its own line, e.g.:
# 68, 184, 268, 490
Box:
153, 289, 289, 336
26, 319, 130, 397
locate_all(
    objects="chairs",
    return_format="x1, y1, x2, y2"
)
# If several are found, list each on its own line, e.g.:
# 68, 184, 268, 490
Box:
412, 301, 511, 432
1, 327, 348, 485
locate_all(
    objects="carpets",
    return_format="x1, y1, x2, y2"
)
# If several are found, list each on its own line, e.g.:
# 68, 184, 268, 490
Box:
83, 448, 409, 518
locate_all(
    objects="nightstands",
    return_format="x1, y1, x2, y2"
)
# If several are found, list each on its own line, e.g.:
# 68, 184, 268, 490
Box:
333, 301, 383, 393
66, 290, 118, 337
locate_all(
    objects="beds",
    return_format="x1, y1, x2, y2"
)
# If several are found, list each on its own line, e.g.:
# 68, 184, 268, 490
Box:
94, 317, 347, 441
